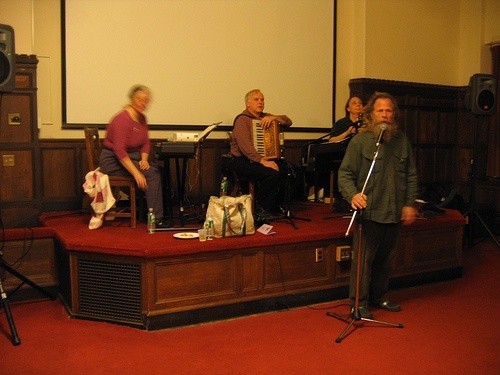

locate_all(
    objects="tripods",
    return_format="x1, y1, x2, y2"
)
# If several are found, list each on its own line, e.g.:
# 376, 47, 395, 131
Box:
462, 115, 500, 249
264, 148, 312, 230
326, 138, 405, 343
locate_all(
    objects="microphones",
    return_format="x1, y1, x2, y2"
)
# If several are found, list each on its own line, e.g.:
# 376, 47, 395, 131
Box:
376, 123, 389, 147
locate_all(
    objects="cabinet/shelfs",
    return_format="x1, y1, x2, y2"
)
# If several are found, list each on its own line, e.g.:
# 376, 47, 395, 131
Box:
348, 78, 489, 185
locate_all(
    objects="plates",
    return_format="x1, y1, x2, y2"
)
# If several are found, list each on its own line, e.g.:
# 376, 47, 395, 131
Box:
173, 232, 199, 239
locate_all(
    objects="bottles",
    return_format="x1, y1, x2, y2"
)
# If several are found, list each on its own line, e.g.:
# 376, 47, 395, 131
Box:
220, 177, 228, 196
147, 208, 155, 234
206, 217, 214, 240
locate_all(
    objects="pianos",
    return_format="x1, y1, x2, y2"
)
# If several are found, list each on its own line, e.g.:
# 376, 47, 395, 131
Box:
153, 141, 196, 227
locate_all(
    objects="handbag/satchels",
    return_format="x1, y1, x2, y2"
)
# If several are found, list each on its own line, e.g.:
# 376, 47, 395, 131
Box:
204, 193, 255, 238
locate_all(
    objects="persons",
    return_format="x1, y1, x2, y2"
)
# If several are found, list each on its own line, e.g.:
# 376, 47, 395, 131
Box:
329, 96, 364, 213
227, 89, 301, 215
338, 93, 418, 319
98, 85, 172, 228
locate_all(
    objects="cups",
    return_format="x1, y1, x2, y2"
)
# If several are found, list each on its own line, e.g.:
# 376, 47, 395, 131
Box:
198, 229, 207, 241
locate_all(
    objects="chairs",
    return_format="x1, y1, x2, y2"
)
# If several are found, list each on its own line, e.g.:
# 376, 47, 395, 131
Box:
301, 139, 329, 203
84, 127, 138, 228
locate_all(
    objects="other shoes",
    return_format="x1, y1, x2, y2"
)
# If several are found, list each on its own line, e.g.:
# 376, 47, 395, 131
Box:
352, 307, 372, 319
368, 299, 400, 311
262, 213, 272, 224
271, 207, 288, 215
156, 221, 172, 228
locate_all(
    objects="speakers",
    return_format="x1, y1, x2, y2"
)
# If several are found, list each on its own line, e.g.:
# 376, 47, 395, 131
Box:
0, 23, 15, 92
465, 74, 497, 115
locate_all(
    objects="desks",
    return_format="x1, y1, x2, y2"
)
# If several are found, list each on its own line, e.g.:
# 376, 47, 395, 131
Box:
149, 150, 196, 228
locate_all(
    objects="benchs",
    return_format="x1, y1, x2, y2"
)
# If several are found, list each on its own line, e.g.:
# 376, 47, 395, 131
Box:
0, 238, 57, 302
